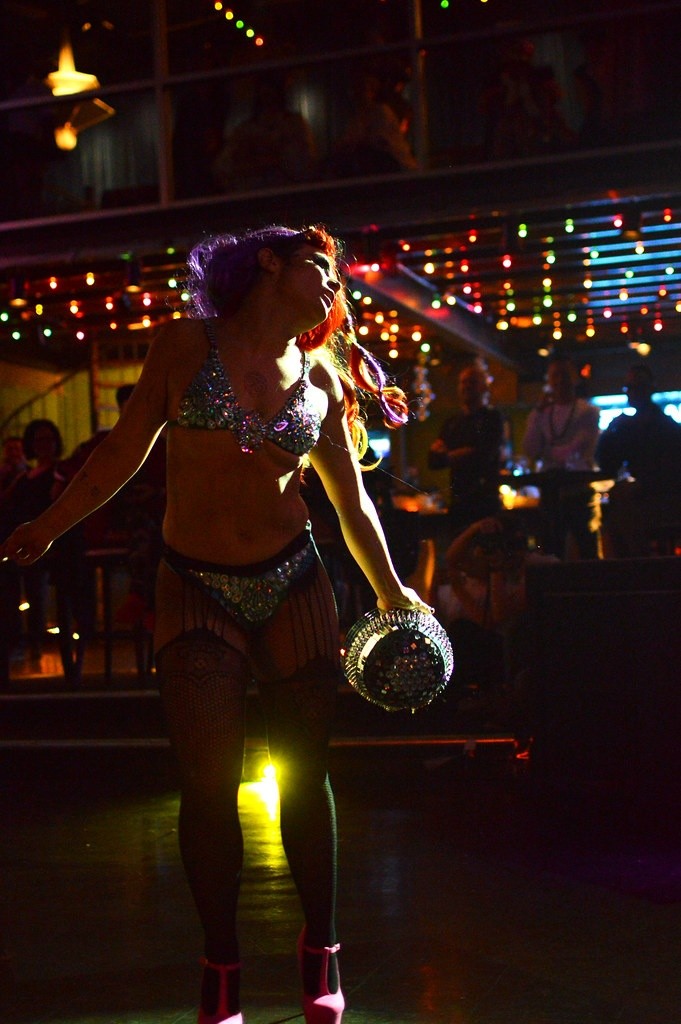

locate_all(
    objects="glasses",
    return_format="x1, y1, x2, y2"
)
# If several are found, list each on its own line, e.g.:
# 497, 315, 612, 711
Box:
621, 383, 642, 393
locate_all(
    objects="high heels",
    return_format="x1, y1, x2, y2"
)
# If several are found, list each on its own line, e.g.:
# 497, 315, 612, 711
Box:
297, 927, 346, 1024
198, 952, 244, 1024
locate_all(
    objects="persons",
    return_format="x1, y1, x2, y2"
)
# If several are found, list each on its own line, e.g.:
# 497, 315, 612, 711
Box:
521, 357, 596, 560
428, 365, 504, 517
0, 227, 436, 1024
0, 384, 166, 658
596, 366, 681, 526
443, 516, 560, 633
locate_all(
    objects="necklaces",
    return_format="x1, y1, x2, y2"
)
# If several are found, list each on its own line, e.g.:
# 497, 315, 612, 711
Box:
548, 400, 576, 437
